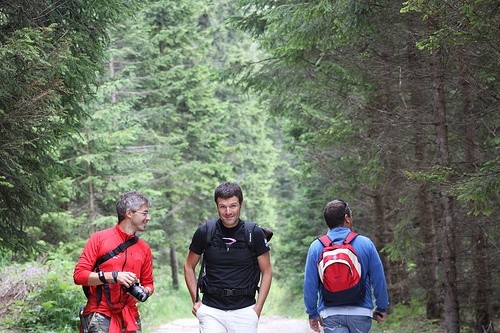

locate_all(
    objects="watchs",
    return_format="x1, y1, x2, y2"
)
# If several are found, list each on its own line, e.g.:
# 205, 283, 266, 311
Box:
112, 271, 118, 284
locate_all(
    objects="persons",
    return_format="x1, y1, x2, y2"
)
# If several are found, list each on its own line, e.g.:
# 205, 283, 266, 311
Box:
303, 200, 388, 333
73, 193, 154, 333
184, 183, 272, 333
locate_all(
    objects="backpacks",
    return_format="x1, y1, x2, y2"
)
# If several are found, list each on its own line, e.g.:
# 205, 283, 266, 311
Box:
316, 231, 365, 307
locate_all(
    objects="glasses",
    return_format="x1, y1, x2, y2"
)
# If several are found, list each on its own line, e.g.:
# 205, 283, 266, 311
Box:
338, 199, 348, 213
135, 211, 149, 217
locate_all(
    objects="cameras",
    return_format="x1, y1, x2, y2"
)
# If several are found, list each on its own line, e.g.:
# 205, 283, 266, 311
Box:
121, 281, 149, 302
